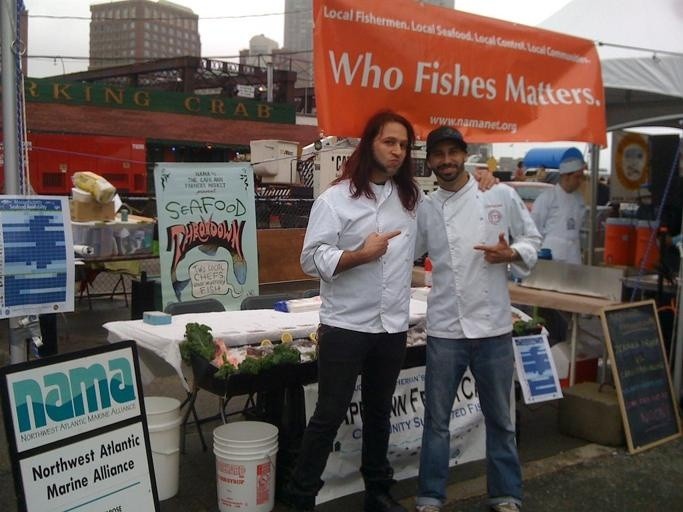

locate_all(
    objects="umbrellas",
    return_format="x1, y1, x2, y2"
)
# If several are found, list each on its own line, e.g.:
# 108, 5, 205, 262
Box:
364, 493, 409, 511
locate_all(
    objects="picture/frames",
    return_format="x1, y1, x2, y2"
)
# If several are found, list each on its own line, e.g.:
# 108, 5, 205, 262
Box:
76, 255, 153, 310
102, 281, 550, 455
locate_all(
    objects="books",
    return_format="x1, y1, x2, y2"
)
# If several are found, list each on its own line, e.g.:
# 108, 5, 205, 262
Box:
559, 380, 623, 448
68, 186, 157, 258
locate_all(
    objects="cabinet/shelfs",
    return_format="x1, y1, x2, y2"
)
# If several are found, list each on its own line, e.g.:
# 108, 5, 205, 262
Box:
658, 227, 668, 244
121, 209, 128, 221
72, 227, 143, 259
424, 255, 433, 288
150, 236, 158, 256
142, 224, 152, 249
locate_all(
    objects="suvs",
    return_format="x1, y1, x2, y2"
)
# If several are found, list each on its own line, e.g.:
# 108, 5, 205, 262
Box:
143, 396, 182, 427
523, 146, 585, 170
211, 419, 282, 512
147, 416, 183, 502
603, 216, 637, 266
633, 220, 659, 271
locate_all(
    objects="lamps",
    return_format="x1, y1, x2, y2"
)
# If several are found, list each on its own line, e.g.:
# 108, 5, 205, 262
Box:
160, 298, 234, 426
239, 293, 305, 415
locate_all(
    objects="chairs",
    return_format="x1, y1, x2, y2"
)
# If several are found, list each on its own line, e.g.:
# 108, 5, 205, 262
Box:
415, 504, 440, 512
491, 502, 520, 512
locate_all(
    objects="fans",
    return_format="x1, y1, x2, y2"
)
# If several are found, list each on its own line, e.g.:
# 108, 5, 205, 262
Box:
600, 299, 682, 455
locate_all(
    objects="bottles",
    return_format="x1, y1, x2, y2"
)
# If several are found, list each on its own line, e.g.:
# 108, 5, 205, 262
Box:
559, 156, 587, 174
426, 126, 466, 152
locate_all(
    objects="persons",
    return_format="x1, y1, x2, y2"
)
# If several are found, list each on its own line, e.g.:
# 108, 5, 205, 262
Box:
513, 161, 526, 181
597, 177, 606, 205
276, 110, 500, 512
530, 148, 586, 341
415, 126, 542, 512
535, 165, 545, 179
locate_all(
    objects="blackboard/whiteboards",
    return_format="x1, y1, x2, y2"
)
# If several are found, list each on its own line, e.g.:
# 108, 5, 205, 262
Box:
249, 128, 442, 228
